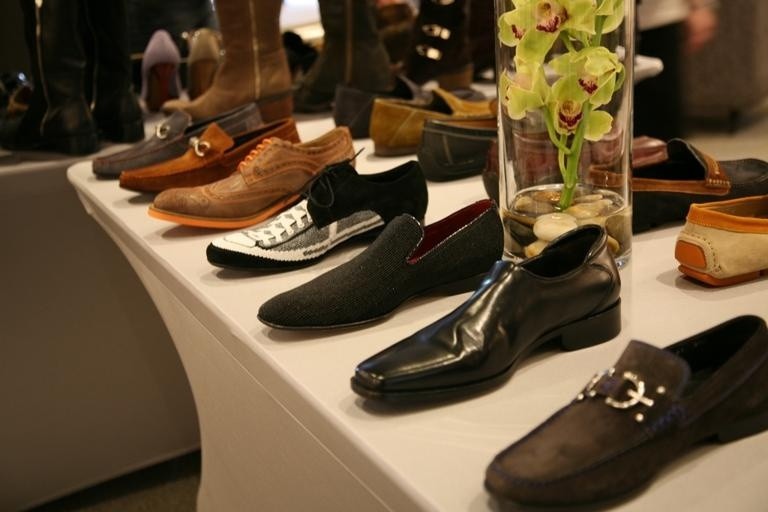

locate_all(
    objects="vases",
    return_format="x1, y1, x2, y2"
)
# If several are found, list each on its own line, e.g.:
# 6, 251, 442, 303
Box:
493, 0, 636, 273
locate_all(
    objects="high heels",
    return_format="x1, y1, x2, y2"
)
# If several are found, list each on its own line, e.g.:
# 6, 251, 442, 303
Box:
140, 29, 180, 112
188, 27, 221, 100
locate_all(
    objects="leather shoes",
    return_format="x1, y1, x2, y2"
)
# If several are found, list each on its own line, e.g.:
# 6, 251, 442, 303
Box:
484, 314, 768, 512
351, 223, 621, 404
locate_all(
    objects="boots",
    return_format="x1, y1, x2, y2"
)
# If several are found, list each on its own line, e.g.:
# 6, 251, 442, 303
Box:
92, 2, 144, 144
0, 2, 110, 155
162, 1, 496, 123
296, 2, 497, 115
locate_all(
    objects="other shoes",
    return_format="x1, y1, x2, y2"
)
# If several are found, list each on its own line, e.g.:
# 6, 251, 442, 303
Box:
119, 118, 301, 195
255, 199, 507, 332
92, 101, 264, 176
369, 85, 497, 157
484, 132, 663, 202
588, 139, 768, 235
417, 116, 502, 183
332, 86, 487, 138
675, 196, 768, 288
206, 146, 431, 272
148, 126, 356, 230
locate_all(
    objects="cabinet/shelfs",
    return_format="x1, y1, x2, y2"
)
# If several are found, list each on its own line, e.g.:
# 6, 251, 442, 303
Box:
0, 115, 768, 511
